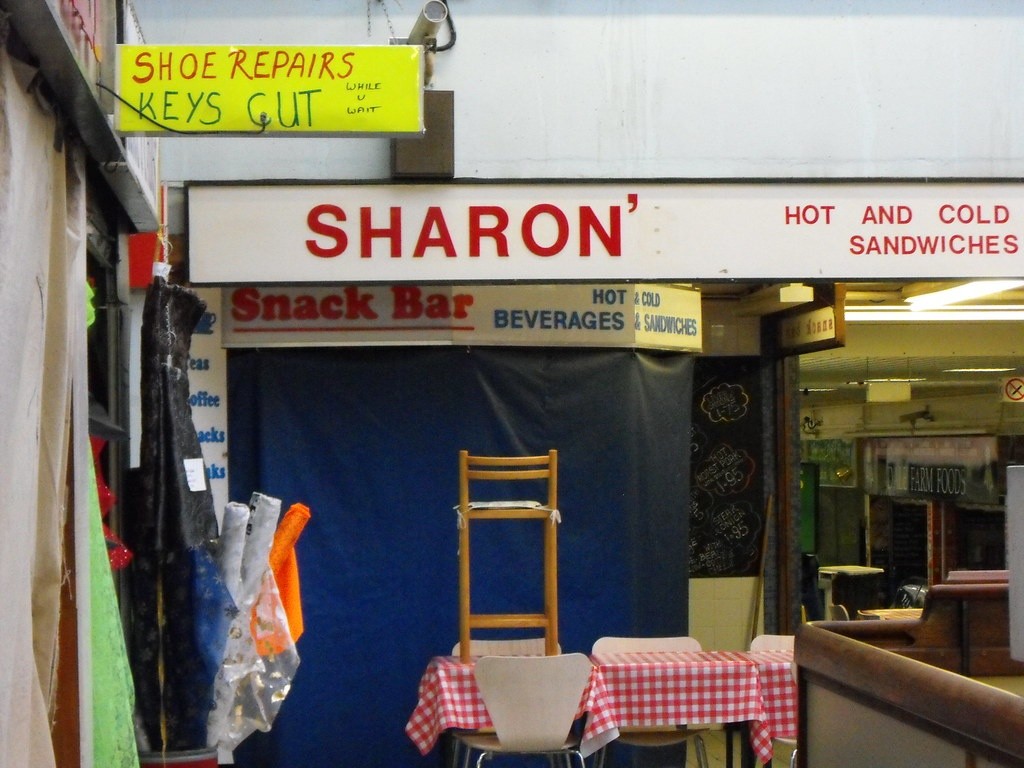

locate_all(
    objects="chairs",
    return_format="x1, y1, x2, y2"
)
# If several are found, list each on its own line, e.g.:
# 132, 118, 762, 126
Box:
452, 635, 796, 768
459, 449, 558, 663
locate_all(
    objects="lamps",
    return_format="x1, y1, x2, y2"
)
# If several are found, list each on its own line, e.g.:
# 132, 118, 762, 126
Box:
901, 278, 1024, 302
733, 280, 813, 316
405, 0, 448, 87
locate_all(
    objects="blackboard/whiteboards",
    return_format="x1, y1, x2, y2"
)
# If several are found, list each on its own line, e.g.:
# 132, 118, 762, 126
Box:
688, 355, 767, 579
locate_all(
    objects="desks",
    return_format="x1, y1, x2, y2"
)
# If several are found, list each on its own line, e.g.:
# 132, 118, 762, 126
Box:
579, 651, 775, 768
405, 653, 598, 768
747, 651, 799, 764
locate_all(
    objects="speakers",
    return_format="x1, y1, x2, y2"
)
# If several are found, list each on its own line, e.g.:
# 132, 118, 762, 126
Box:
391, 91, 454, 177
